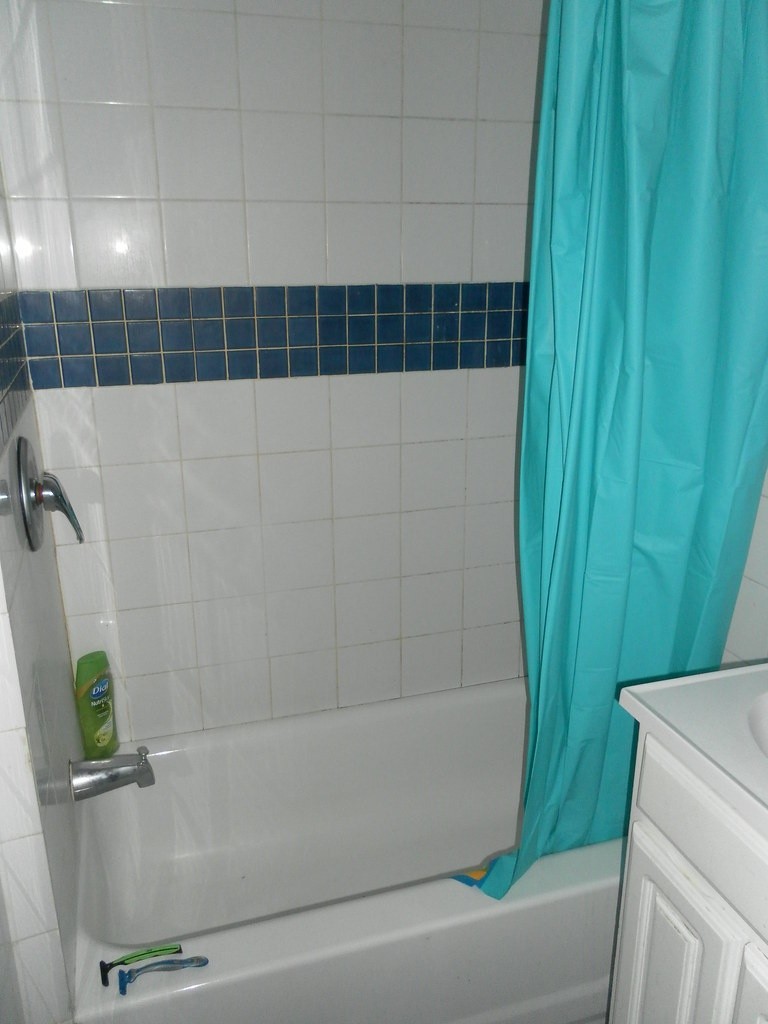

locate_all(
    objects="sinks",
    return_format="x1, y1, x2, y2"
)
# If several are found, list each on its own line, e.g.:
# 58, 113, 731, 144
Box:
619, 656, 768, 829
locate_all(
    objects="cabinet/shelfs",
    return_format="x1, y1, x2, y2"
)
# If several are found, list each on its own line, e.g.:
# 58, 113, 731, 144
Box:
608, 724, 768, 1024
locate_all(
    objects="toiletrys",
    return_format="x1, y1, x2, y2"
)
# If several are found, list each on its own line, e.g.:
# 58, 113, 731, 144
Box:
75, 649, 122, 760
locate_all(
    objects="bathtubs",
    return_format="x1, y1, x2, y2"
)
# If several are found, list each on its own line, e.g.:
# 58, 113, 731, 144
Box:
73, 667, 626, 1023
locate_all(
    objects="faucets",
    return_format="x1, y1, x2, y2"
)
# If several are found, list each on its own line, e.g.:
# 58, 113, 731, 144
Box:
70, 746, 155, 800
